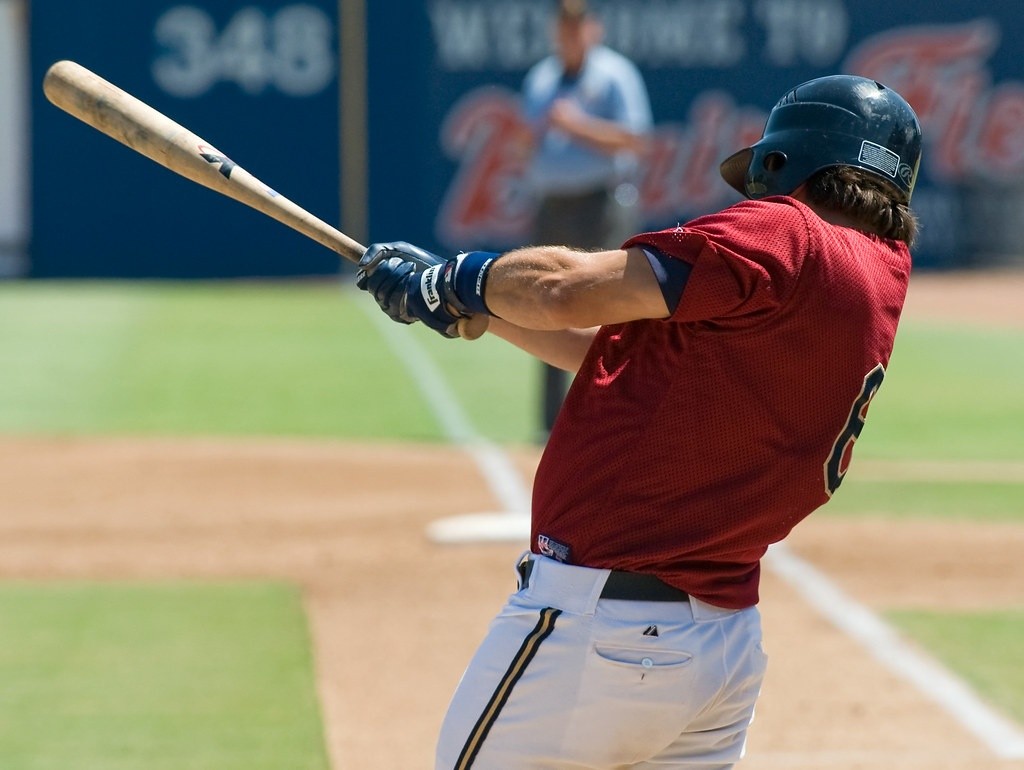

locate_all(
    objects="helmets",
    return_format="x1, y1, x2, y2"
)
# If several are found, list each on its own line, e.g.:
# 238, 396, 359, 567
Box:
720, 75, 923, 212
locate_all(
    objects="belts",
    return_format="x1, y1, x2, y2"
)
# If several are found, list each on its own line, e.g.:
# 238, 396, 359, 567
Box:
516, 561, 690, 603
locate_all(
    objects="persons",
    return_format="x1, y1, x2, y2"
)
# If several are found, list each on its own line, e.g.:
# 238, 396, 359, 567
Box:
524, 1, 656, 443
357, 76, 924, 770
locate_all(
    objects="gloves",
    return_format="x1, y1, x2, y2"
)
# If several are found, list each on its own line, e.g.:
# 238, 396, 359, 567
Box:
356, 241, 471, 339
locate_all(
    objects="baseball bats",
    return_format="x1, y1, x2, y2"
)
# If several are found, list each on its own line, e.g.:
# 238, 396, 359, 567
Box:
42, 58, 491, 342
